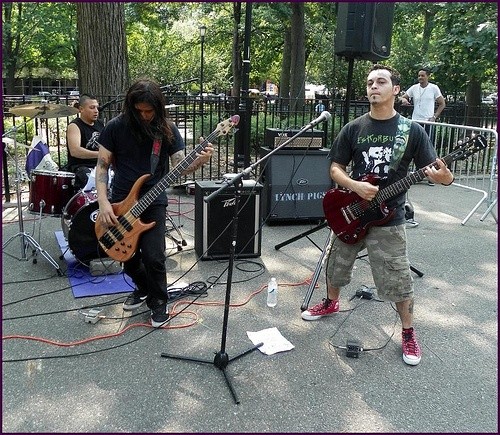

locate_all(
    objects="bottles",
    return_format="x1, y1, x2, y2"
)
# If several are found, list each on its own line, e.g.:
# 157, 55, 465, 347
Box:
266, 277, 278, 307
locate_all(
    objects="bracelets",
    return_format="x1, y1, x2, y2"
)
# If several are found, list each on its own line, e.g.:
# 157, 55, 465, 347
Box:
434, 116, 436, 119
441, 178, 455, 186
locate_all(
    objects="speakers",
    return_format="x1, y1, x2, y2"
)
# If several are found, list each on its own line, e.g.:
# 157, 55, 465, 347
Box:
335, 2, 394, 62
195, 181, 264, 261
259, 146, 336, 224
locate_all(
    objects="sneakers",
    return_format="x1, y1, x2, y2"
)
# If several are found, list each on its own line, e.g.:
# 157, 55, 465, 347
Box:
150, 304, 169, 327
401, 327, 421, 365
301, 297, 340, 320
122, 291, 148, 310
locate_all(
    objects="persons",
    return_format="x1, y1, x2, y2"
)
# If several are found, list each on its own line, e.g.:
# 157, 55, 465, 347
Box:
315, 100, 325, 112
301, 65, 456, 365
399, 69, 447, 187
95, 79, 214, 328
66, 93, 104, 192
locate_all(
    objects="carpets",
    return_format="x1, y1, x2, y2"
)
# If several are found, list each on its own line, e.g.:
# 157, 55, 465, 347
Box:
54, 230, 138, 299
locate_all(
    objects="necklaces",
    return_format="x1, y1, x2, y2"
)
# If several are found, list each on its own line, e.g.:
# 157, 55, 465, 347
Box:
419, 87, 425, 97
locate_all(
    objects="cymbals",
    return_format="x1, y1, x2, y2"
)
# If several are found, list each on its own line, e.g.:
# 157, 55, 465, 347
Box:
9, 103, 80, 118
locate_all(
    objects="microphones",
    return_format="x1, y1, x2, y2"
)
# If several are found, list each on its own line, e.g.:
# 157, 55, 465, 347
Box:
305, 111, 332, 130
98, 102, 109, 112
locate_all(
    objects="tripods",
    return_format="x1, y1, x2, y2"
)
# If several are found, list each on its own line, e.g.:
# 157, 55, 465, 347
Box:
1, 107, 64, 277
160, 126, 307, 406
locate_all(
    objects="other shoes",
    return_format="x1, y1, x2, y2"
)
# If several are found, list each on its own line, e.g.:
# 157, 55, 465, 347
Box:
427, 181, 435, 186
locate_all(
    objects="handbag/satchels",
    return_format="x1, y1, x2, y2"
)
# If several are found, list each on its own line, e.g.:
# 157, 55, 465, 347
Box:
24, 135, 59, 173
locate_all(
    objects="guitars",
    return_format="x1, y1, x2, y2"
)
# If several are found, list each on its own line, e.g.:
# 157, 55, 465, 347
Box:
93, 114, 240, 260
322, 133, 487, 244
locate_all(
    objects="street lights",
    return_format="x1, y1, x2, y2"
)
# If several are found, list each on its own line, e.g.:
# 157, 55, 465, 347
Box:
56, 80, 60, 89
198, 23, 207, 116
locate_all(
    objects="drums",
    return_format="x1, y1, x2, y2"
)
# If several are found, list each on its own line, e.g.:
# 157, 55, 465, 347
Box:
60, 189, 109, 267
27, 169, 77, 217
